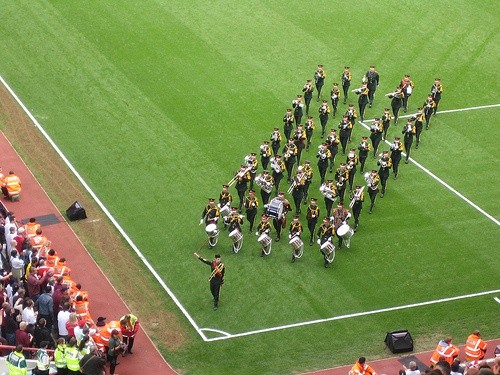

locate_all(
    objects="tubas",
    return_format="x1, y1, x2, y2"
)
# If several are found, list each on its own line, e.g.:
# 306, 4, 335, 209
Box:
270, 157, 281, 173
319, 183, 337, 201
365, 172, 373, 185
244, 156, 254, 166
256, 175, 273, 193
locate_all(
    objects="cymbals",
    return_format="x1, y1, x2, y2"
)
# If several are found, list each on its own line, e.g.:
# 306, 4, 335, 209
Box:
306, 210, 312, 221
249, 201, 252, 209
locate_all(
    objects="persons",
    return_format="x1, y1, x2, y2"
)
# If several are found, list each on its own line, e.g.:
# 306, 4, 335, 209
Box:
314, 216, 336, 267
397, 369, 406, 375
403, 361, 419, 375
255, 212, 274, 256
200, 198, 221, 247
194, 251, 226, 312
233, 65, 443, 229
222, 207, 247, 254
329, 202, 353, 249
425, 344, 500, 375
0, 167, 22, 199
264, 193, 293, 240
430, 336, 461, 365
464, 329, 488, 362
285, 213, 307, 261
348, 356, 378, 375
217, 184, 233, 230
306, 198, 320, 246
243, 190, 259, 234
1, 198, 140, 375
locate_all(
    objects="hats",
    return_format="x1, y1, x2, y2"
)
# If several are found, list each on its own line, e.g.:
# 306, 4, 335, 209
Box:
327, 179, 333, 183
223, 183, 230, 188
251, 152, 257, 156
263, 169, 269, 174
337, 201, 344, 206
304, 159, 310, 164
260, 213, 268, 219
275, 154, 282, 158
296, 171, 301, 175
371, 169, 377, 173
263, 140, 270, 145
355, 185, 362, 189
273, 64, 440, 155
323, 216, 330, 223
208, 198, 215, 202
240, 164, 246, 168
61, 285, 69, 291
311, 197, 317, 201
278, 191, 284, 194
339, 162, 347, 167
293, 214, 299, 219
215, 253, 220, 258
231, 206, 238, 212
98, 316, 106, 322
248, 189, 255, 193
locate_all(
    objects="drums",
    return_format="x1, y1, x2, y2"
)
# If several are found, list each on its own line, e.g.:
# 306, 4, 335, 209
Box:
229, 228, 243, 243
289, 236, 303, 250
320, 241, 335, 255
267, 199, 283, 220
258, 233, 272, 247
205, 223, 220, 238
336, 224, 354, 240
219, 205, 232, 216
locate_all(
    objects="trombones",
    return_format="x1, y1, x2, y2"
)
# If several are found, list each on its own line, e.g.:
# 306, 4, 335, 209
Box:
227, 166, 252, 187
349, 186, 365, 208
287, 172, 305, 194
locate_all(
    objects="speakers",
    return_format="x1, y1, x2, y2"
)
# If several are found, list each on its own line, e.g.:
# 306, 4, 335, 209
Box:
67, 201, 87, 221
385, 329, 415, 354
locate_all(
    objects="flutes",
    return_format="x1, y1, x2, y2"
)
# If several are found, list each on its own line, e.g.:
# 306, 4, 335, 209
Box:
294, 69, 439, 123
352, 86, 365, 92
385, 92, 400, 96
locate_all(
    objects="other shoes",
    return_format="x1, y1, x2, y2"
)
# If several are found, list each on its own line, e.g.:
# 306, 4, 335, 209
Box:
214, 305, 218, 311
208, 93, 437, 267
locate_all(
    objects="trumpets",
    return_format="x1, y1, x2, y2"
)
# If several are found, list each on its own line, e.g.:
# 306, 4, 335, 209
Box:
261, 112, 409, 187
